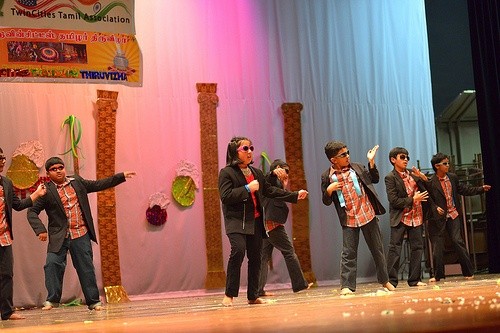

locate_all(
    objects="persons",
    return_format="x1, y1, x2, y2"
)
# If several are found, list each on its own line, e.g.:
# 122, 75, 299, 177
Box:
0, 147, 47, 320
383, 147, 432, 287
27, 157, 137, 311
426, 153, 491, 283
321, 142, 395, 295
219, 137, 308, 305
256, 159, 313, 296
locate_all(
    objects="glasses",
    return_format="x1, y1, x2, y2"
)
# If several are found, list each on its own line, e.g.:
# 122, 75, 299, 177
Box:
335, 150, 349, 157
0, 157, 6, 160
236, 146, 254, 152
400, 155, 409, 161
285, 169, 289, 174
437, 162, 450, 165
49, 166, 64, 171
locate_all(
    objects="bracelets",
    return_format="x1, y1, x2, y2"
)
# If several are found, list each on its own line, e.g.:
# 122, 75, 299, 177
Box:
245, 184, 251, 193
